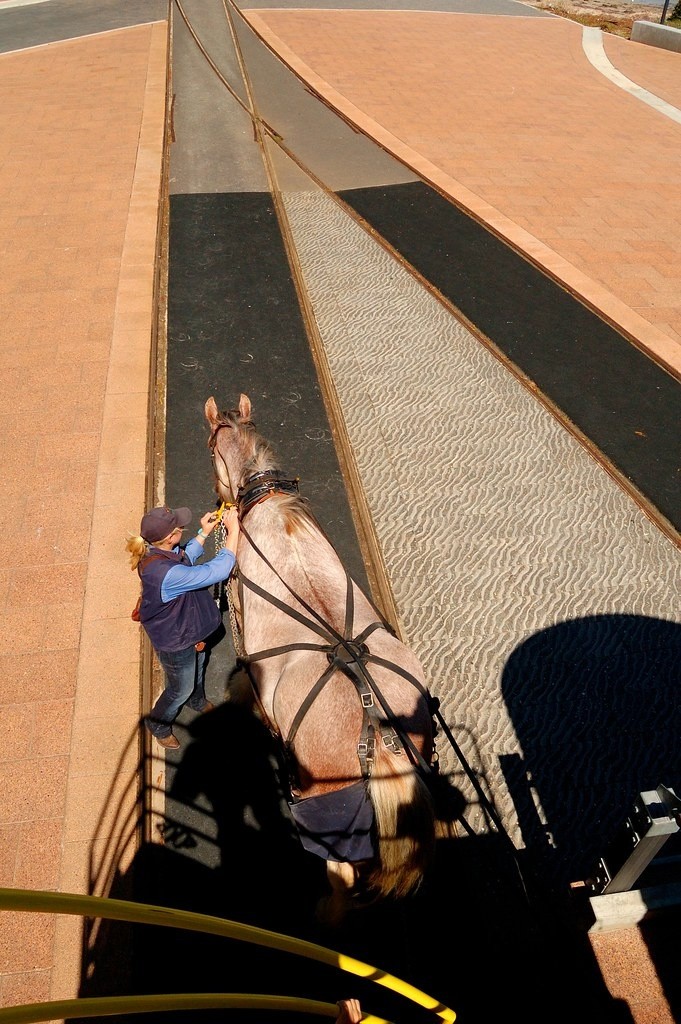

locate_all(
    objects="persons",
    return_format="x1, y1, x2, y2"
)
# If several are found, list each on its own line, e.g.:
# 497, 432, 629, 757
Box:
123, 506, 243, 745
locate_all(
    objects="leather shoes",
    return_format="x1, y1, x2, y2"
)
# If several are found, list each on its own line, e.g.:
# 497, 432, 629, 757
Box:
199, 702, 213, 714
152, 734, 180, 751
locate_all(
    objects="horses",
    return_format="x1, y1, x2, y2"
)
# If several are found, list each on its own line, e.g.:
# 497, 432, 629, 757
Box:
203, 394, 460, 932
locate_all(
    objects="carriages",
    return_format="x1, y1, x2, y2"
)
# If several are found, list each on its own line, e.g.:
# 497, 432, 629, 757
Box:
4, 397, 679, 1024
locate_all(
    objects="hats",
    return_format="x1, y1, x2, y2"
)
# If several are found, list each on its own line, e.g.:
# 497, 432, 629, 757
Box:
139, 506, 193, 545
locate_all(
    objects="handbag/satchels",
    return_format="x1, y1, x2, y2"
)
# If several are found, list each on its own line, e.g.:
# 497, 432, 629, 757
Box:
130, 597, 142, 622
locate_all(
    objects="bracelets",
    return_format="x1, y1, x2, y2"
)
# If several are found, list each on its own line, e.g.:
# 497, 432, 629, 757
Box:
198, 528, 211, 539
